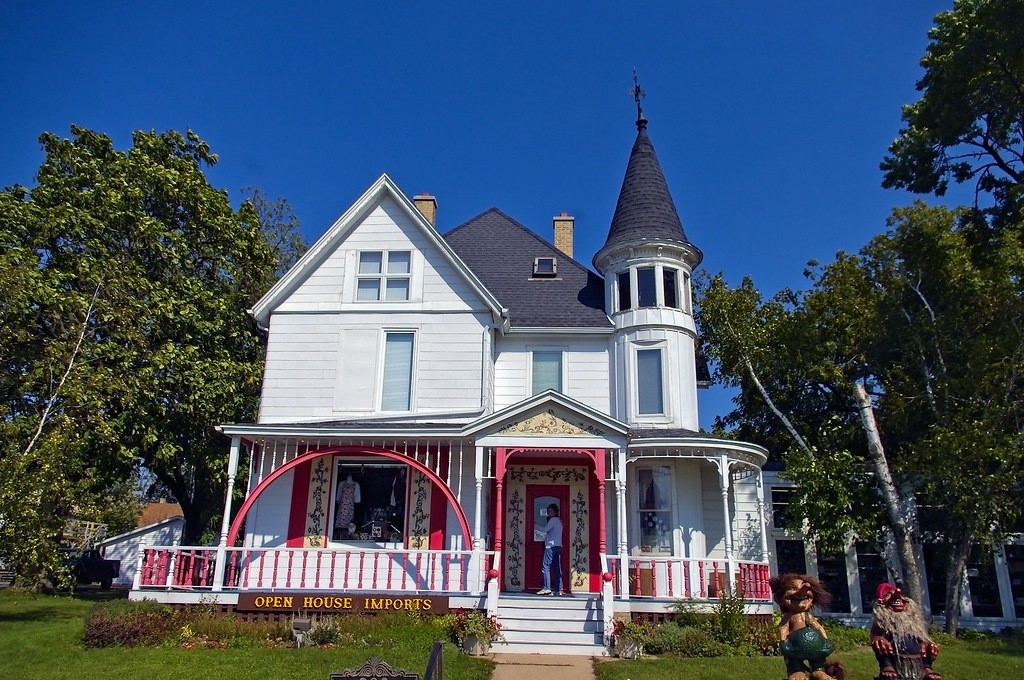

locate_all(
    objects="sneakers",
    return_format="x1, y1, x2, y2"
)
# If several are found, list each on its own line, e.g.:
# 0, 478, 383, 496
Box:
536, 588, 552, 594
550, 590, 563, 596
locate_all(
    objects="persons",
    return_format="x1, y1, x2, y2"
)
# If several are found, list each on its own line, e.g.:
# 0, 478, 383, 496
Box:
870, 584, 941, 680
534, 504, 564, 595
769, 574, 835, 680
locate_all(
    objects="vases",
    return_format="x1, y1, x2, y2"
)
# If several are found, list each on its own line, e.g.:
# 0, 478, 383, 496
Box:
618, 637, 643, 659
463, 635, 489, 656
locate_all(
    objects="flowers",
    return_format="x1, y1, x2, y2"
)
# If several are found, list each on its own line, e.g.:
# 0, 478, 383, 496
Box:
614, 613, 655, 638
451, 607, 509, 648
